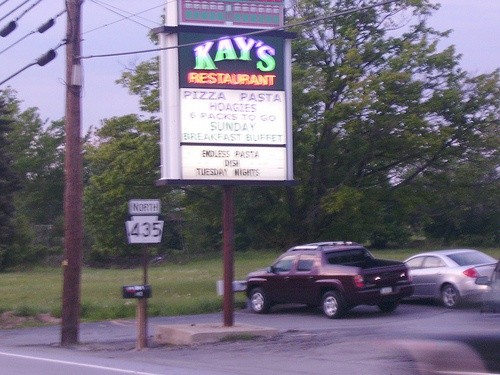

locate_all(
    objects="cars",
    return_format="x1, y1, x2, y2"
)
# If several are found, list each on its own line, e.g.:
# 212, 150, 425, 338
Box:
475, 260, 500, 313
399, 248, 500, 308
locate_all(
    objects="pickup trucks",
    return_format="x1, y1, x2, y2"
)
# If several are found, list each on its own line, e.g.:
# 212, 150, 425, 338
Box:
241, 240, 415, 318
